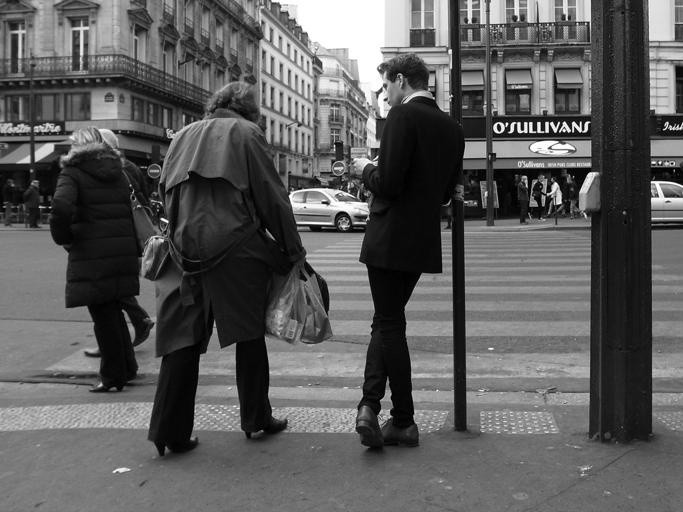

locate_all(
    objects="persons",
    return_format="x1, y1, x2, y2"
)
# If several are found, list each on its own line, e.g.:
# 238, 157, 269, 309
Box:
22, 180, 41, 229
466, 172, 587, 226
1, 177, 18, 227
47, 123, 148, 395
347, 52, 466, 452
80, 128, 157, 359
440, 199, 453, 230
143, 80, 308, 459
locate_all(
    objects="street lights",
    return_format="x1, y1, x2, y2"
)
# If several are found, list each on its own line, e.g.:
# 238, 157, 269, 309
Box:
28, 49, 39, 182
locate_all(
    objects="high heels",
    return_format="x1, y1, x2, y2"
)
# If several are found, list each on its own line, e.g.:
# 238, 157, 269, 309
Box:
154, 437, 197, 456
245, 416, 287, 438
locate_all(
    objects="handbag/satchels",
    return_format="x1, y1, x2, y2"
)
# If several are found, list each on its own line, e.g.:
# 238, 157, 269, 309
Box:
141, 235, 170, 281
121, 168, 158, 256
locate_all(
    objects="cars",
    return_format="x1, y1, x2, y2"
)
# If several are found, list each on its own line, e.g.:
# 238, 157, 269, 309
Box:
651, 181, 683, 223
288, 187, 370, 231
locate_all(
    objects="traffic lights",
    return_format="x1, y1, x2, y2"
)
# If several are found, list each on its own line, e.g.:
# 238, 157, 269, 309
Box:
334, 140, 344, 160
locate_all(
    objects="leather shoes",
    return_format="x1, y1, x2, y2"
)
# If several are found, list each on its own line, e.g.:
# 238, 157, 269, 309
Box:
132, 317, 154, 346
381, 418, 418, 448
89, 384, 122, 392
84, 348, 101, 357
355, 406, 384, 449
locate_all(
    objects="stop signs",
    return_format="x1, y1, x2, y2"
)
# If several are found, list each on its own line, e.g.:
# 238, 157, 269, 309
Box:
147, 163, 161, 178
332, 162, 346, 176
